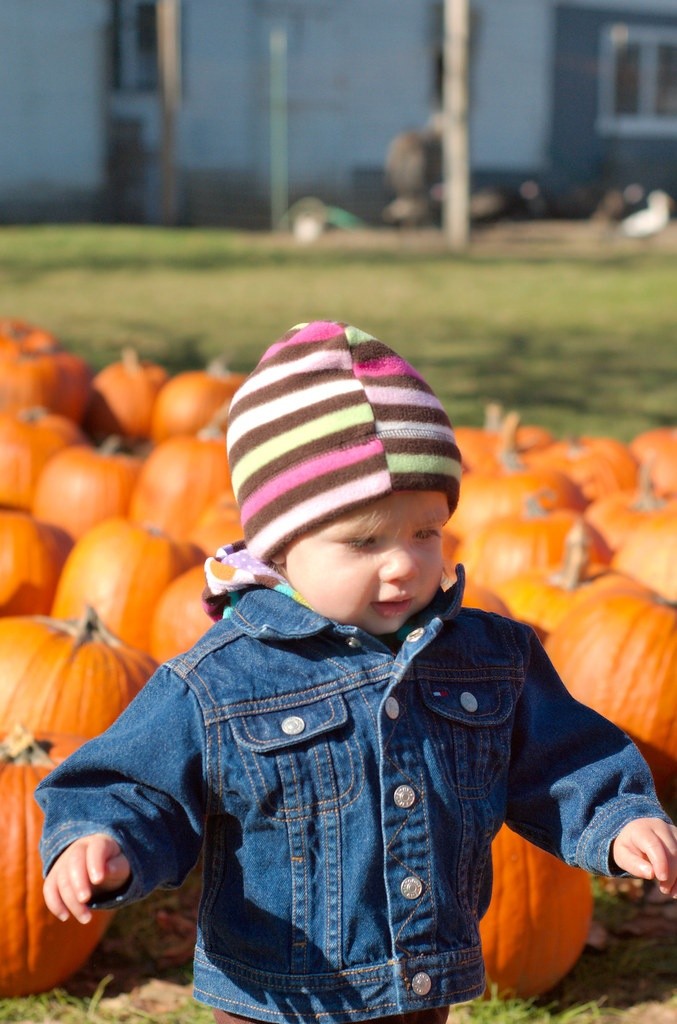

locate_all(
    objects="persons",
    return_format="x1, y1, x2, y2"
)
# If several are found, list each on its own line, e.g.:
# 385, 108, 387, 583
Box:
30, 317, 677, 1024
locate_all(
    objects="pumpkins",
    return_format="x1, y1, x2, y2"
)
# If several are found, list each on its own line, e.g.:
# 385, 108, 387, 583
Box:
0, 316, 249, 1001
431, 402, 677, 1002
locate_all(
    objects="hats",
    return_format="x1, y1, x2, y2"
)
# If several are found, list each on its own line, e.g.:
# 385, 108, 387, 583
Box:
227, 322, 461, 564
